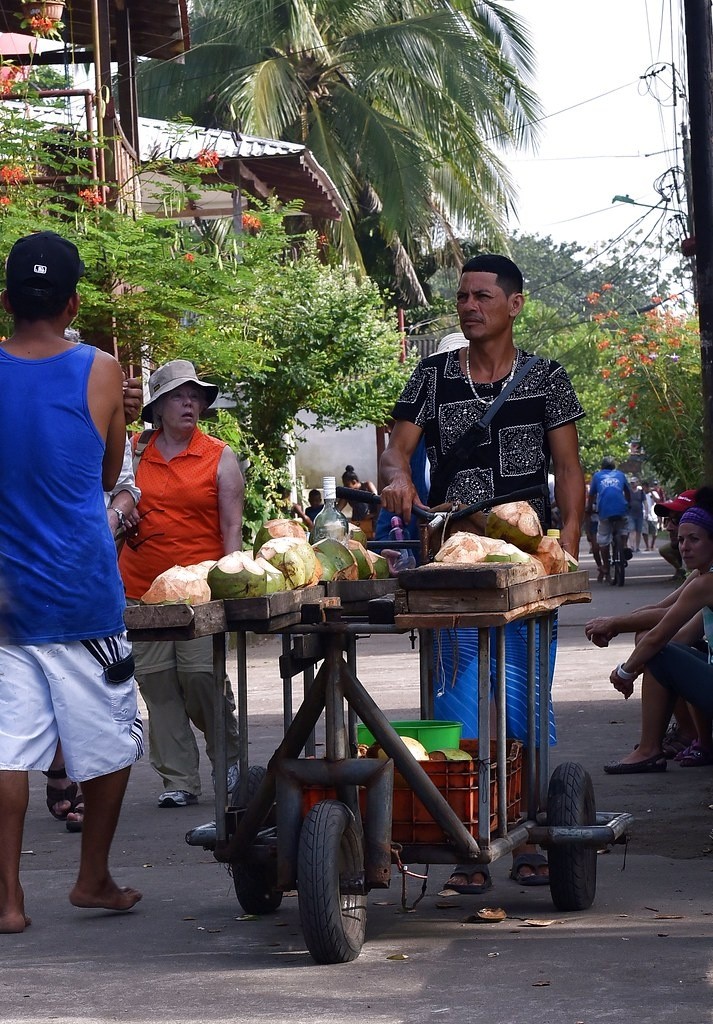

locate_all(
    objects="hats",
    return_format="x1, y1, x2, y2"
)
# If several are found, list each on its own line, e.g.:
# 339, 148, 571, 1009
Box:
142, 360, 219, 423
7, 231, 84, 296
603, 456, 613, 466
654, 490, 697, 517
630, 477, 639, 482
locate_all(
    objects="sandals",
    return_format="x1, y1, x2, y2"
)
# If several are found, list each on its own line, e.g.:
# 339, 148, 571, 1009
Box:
42, 766, 78, 819
66, 795, 83, 831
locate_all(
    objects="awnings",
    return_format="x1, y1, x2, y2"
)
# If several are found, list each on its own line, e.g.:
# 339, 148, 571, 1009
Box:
0, 97, 350, 227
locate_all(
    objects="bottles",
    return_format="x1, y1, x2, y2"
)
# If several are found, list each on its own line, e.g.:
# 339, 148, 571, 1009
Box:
390, 515, 416, 573
312, 476, 349, 545
547, 529, 560, 542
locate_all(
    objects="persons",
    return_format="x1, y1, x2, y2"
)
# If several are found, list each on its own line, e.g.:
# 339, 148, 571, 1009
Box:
379, 255, 587, 894
0, 229, 245, 933
547, 457, 673, 583
274, 465, 381, 533
584, 487, 713, 772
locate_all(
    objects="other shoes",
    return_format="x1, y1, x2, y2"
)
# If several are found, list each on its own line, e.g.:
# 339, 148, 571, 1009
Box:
634, 722, 694, 758
604, 753, 667, 774
675, 739, 713, 767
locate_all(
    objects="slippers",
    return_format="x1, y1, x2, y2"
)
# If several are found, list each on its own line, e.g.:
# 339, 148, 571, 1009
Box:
510, 854, 551, 884
444, 862, 493, 893
624, 548, 633, 560
597, 566, 610, 575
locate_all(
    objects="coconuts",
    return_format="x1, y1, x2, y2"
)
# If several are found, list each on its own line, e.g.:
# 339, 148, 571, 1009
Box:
364, 737, 475, 761
139, 519, 389, 607
435, 498, 580, 578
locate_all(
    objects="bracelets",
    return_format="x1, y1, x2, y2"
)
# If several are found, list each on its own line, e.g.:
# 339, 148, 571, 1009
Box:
617, 663, 634, 680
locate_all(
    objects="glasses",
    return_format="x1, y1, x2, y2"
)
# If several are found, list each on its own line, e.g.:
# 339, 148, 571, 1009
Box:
125, 509, 165, 552
664, 513, 683, 527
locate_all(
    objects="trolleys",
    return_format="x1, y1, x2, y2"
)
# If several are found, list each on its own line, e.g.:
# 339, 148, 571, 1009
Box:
184, 486, 637, 965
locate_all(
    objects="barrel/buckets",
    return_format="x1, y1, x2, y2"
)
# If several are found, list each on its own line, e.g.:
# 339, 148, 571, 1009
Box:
357, 721, 464, 754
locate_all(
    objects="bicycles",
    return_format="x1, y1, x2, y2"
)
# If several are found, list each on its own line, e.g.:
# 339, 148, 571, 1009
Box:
585, 506, 633, 587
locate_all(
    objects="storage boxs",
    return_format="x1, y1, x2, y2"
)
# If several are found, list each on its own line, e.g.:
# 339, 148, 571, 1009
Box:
301, 740, 523, 848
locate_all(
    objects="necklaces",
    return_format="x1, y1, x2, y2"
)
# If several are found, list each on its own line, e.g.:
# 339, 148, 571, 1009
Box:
465, 345, 520, 406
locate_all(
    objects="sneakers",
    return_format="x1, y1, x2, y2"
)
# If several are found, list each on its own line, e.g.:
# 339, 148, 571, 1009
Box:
159, 790, 198, 806
211, 762, 237, 794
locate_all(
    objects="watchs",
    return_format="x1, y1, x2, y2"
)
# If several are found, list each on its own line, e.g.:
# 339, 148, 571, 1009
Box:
107, 507, 125, 523
